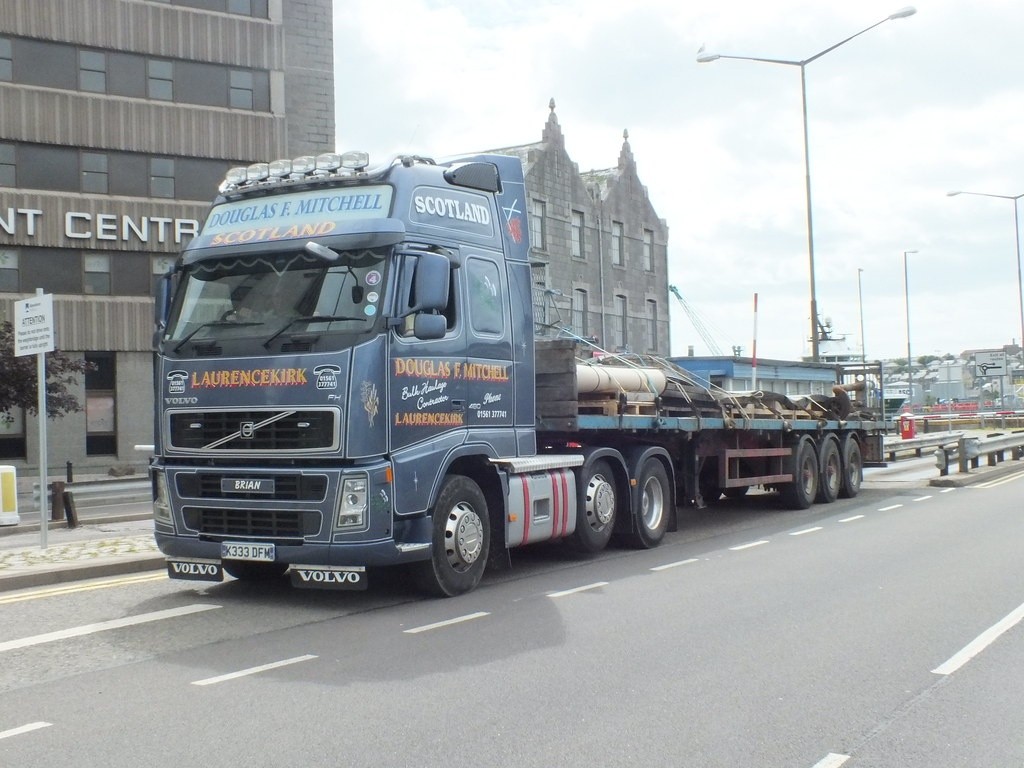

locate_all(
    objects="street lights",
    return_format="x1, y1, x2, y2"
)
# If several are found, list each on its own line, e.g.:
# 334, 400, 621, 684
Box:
905, 249, 919, 417
947, 191, 1024, 355
697, 5, 918, 362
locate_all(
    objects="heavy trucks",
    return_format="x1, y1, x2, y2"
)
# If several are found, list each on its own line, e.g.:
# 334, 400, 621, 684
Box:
134, 152, 899, 605
